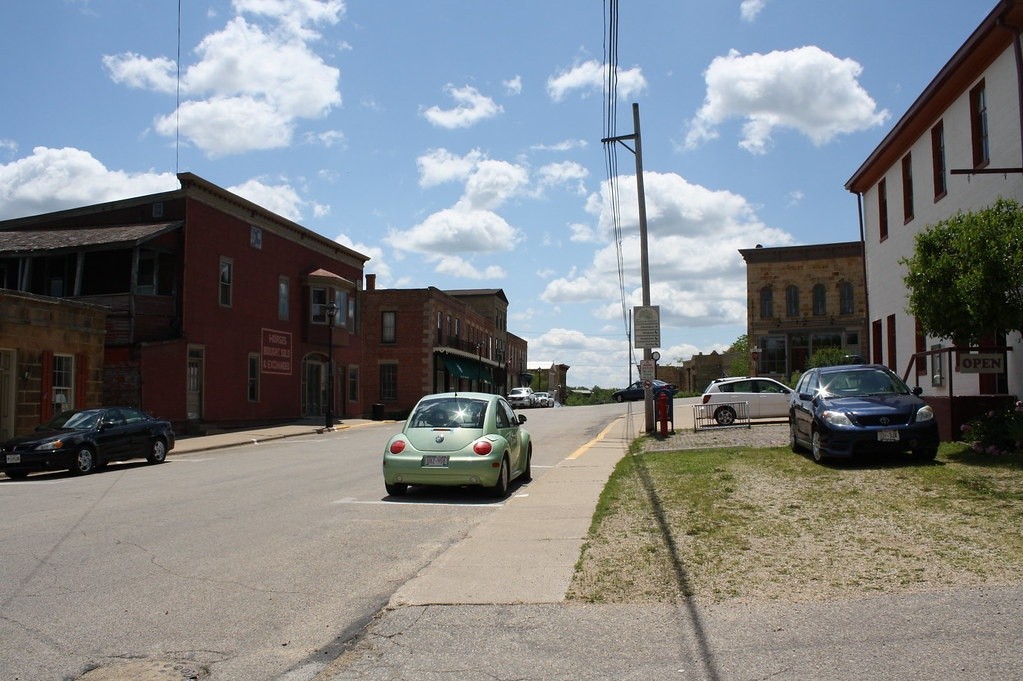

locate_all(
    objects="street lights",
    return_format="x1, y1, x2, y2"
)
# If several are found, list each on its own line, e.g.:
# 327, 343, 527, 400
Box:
497, 354, 502, 395
325, 299, 340, 426
478, 339, 484, 392
538, 368, 541, 392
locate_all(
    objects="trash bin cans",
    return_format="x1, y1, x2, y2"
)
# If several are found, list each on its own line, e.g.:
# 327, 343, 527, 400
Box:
372, 404, 385, 420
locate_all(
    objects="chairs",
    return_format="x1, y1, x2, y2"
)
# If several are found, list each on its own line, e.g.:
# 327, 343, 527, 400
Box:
82, 416, 92, 427
432, 409, 449, 427
470, 405, 482, 427
445, 419, 461, 428
827, 387, 843, 397
728, 384, 734, 392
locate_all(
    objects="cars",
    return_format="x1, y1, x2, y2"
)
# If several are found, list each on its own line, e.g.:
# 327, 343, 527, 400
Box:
789, 365, 939, 465
534, 392, 554, 408
697, 377, 794, 426
506, 387, 536, 408
0, 406, 175, 479
383, 392, 533, 496
612, 380, 678, 403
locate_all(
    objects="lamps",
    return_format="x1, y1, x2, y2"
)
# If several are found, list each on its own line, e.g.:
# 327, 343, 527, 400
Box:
51, 393, 66, 404
